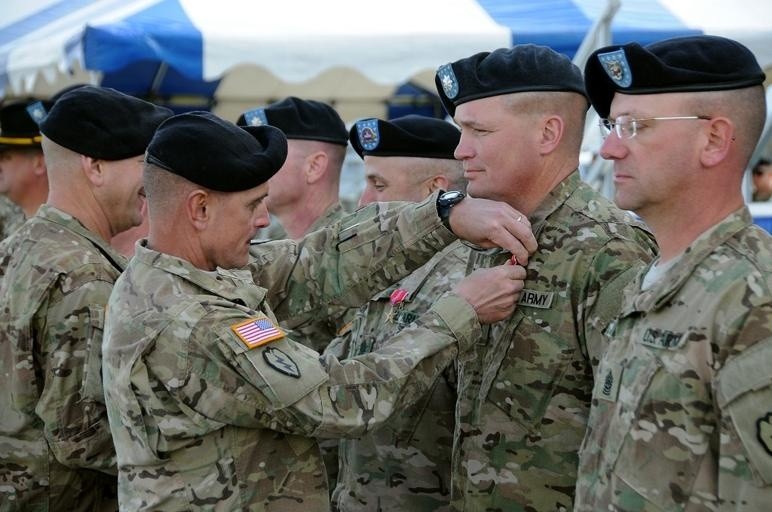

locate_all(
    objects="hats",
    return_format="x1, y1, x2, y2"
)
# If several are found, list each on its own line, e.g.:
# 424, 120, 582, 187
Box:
585, 35, 766, 128
144, 110, 288, 191
1, 98, 54, 149
348, 115, 461, 161
237, 98, 348, 147
38, 84, 175, 160
435, 44, 591, 118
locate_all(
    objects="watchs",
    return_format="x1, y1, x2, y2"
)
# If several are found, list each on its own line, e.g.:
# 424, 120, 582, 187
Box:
436, 189, 465, 234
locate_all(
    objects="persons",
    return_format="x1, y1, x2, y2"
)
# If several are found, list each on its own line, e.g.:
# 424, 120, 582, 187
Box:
317, 115, 470, 512
746, 153, 770, 206
232, 96, 345, 250
0, 83, 173, 509
571, 32, 771, 509
101, 111, 541, 512
425, 48, 660, 508
104, 209, 147, 258
0, 93, 58, 225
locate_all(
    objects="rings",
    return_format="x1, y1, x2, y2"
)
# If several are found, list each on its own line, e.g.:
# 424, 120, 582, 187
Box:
513, 213, 524, 225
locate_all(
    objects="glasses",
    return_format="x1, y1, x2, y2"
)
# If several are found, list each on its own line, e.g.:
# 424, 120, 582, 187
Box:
598, 116, 712, 139
